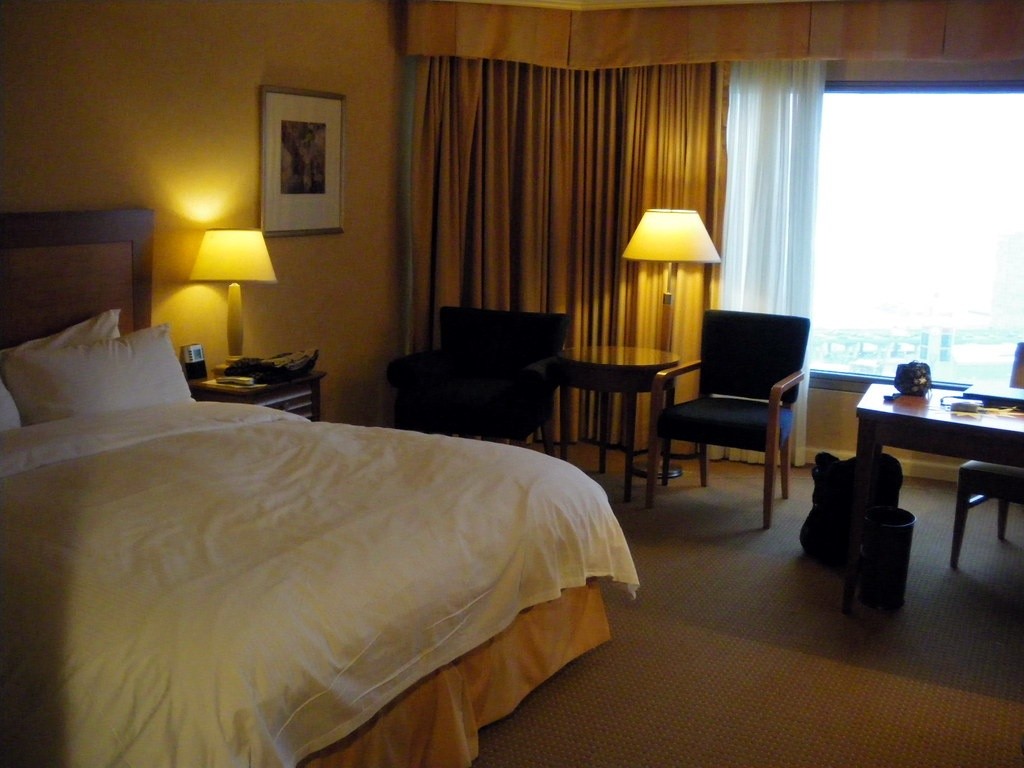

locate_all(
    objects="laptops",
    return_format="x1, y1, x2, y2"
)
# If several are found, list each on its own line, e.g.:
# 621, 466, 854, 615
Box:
963, 385, 1024, 411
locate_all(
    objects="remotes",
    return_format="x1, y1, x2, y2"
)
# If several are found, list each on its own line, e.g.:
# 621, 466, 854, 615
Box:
216, 376, 254, 384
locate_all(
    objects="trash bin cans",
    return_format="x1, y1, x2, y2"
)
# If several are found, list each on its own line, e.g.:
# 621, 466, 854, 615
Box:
854, 503, 919, 613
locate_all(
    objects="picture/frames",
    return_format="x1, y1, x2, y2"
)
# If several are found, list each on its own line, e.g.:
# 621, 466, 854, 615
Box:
258, 83, 347, 239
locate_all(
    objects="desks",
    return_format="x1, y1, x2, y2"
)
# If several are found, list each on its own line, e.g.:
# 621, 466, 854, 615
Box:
554, 345, 680, 503
840, 382, 1024, 616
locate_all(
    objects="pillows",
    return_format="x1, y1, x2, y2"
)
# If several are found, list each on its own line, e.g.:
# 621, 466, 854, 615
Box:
0, 309, 122, 361
0, 322, 197, 430
0, 380, 21, 432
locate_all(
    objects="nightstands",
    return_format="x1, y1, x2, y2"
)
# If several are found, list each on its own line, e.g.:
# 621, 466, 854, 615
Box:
187, 369, 329, 423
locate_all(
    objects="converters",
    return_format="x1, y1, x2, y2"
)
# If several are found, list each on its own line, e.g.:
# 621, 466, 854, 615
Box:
950, 399, 984, 412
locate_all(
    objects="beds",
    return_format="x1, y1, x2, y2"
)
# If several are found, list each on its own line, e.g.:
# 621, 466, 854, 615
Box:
0, 205, 641, 768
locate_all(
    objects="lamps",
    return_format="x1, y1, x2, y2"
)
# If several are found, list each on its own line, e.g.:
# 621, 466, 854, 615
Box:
622, 207, 721, 479
189, 228, 278, 375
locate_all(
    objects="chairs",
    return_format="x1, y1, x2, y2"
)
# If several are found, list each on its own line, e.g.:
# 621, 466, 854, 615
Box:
948, 342, 1024, 569
643, 309, 810, 530
385, 305, 573, 459
